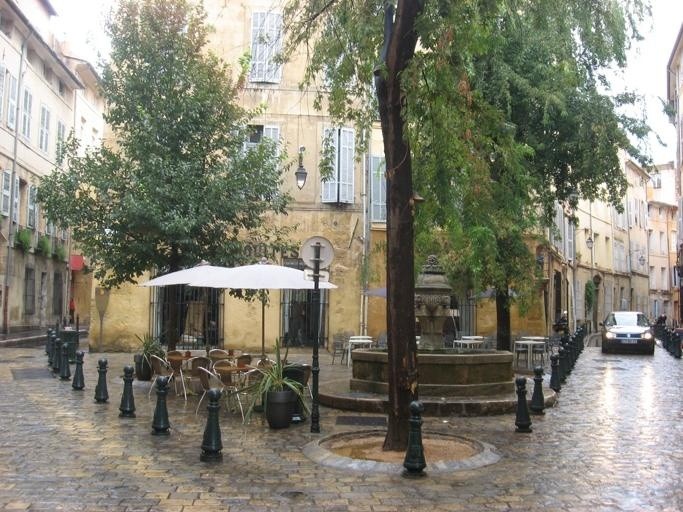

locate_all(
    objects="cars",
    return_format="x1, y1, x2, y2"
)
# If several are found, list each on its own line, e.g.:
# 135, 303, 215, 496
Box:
598, 311, 657, 357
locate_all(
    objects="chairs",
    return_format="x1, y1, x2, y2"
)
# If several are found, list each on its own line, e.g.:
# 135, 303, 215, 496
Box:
332, 333, 380, 366
148, 349, 309, 423
452, 335, 555, 370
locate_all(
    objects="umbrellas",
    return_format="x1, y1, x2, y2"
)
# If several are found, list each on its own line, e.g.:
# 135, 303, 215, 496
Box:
467, 285, 518, 298
361, 287, 387, 297
135, 259, 233, 378
188, 256, 339, 406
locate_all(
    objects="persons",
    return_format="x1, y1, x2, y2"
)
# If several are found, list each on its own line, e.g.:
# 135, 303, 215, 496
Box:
557, 310, 570, 334
69, 297, 75, 324
285, 299, 306, 348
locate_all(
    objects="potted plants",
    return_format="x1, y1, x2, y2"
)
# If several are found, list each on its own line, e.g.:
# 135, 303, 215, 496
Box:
133, 327, 171, 380
230, 338, 311, 429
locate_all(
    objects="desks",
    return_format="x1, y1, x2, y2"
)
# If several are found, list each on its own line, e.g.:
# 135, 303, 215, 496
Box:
348, 340, 374, 368
351, 335, 373, 347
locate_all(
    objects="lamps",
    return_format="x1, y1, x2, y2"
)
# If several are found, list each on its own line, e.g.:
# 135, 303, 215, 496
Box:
295, 146, 307, 191
628, 249, 645, 266
575, 226, 594, 250
